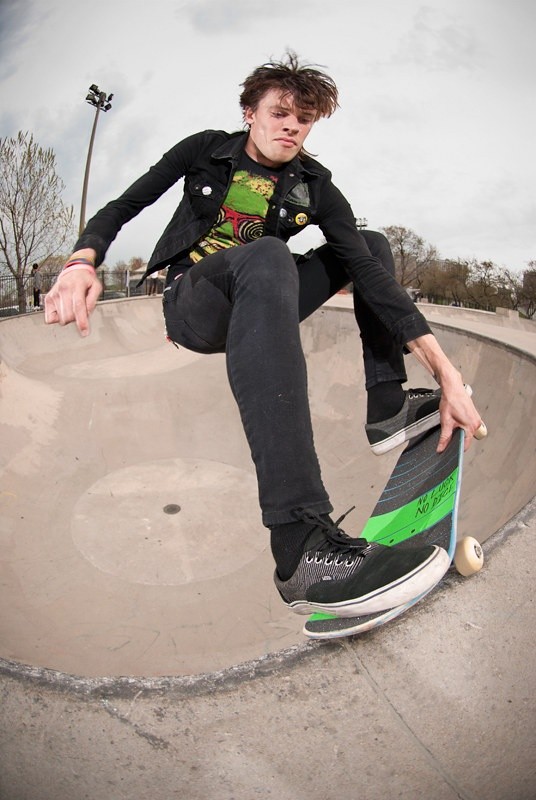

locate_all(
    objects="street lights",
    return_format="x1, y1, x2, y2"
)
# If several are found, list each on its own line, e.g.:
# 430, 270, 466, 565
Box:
76, 82, 114, 237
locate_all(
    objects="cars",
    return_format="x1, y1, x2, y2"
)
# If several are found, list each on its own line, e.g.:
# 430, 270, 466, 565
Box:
99, 289, 127, 301
0, 305, 34, 317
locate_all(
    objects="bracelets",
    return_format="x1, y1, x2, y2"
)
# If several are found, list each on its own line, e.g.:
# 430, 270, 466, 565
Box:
57, 255, 96, 282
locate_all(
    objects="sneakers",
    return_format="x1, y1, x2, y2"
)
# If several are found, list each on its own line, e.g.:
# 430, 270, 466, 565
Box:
274, 505, 449, 618
365, 384, 473, 457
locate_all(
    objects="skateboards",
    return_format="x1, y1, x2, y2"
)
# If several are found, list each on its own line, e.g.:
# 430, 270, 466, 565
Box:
302, 383, 488, 640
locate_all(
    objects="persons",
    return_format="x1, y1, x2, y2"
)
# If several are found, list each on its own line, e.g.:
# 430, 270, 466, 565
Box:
44, 50, 483, 618
33, 263, 42, 310
148, 271, 157, 296
